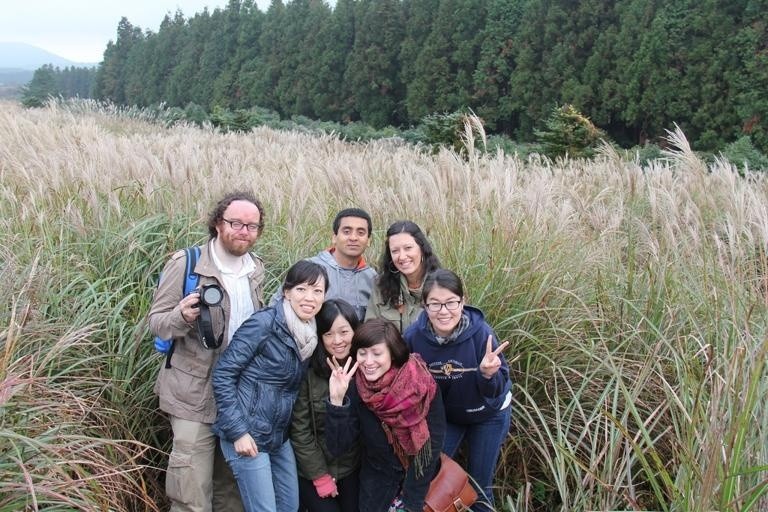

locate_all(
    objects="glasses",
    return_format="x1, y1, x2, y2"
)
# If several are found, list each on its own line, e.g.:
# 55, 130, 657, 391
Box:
222, 217, 262, 233
425, 298, 462, 313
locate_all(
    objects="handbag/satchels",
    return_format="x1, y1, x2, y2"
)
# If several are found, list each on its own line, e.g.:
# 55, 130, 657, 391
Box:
423, 454, 479, 512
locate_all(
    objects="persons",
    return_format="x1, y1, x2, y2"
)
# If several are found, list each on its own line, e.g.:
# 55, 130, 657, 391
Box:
364, 218, 443, 333
286, 298, 360, 512
150, 192, 263, 512
404, 270, 514, 512
211, 258, 331, 512
269, 208, 379, 320
325, 316, 444, 512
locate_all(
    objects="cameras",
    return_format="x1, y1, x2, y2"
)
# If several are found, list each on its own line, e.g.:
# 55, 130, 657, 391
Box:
189, 285, 223, 307
